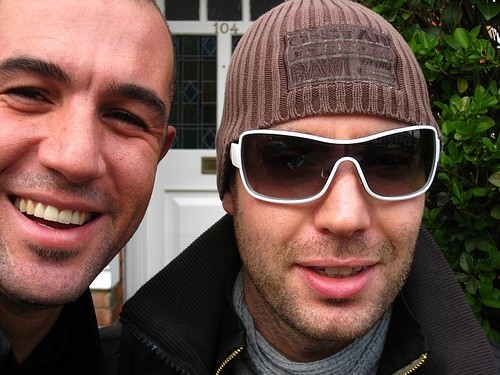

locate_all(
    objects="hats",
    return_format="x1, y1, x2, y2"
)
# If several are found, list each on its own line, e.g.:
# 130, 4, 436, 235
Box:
215, 0, 442, 201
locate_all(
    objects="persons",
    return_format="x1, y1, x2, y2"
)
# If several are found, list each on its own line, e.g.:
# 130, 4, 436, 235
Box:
0, 1, 176, 375
96, 0, 497, 375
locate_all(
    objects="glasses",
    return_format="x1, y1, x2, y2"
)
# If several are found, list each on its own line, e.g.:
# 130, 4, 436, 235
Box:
230, 125, 439, 204
